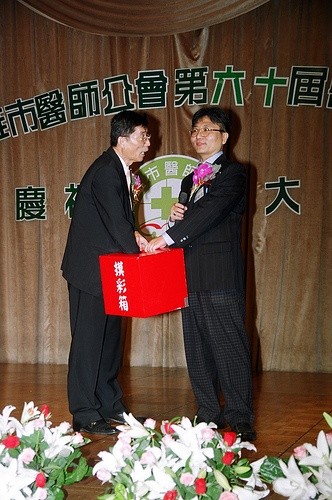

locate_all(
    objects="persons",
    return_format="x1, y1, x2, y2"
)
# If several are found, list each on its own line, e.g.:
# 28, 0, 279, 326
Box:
144, 107, 256, 441
59, 110, 151, 435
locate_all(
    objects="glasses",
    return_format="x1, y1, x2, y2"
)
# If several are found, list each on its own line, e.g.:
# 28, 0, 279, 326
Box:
122, 134, 151, 142
188, 127, 225, 137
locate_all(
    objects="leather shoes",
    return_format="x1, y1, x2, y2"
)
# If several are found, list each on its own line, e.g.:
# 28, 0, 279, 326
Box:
75, 420, 119, 435
107, 413, 144, 426
231, 422, 257, 442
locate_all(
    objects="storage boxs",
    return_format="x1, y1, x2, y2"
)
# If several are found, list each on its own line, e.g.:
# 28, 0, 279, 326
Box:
99, 248, 189, 318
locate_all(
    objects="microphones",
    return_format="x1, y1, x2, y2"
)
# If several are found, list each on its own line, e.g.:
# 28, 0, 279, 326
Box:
175, 192, 187, 225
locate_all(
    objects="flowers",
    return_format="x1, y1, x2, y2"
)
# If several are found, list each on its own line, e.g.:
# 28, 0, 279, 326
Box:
193, 163, 222, 188
92, 412, 271, 500
132, 173, 144, 203
0, 400, 94, 499
260, 412, 332, 500
